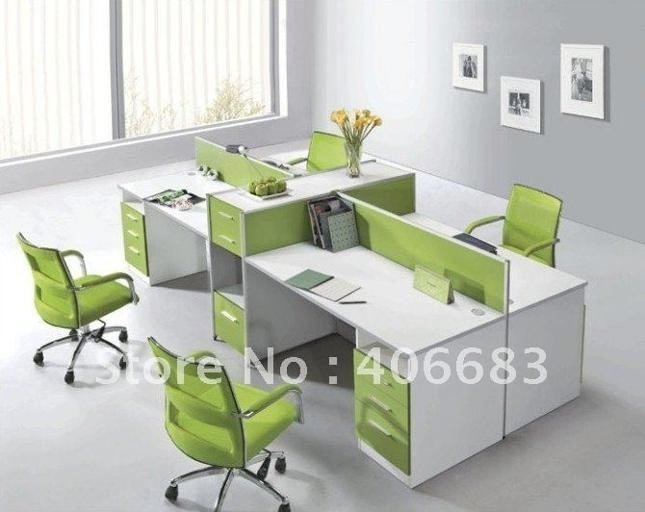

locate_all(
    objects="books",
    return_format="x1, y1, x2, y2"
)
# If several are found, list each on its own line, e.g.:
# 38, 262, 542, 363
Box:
143, 189, 205, 211
307, 196, 360, 253
284, 269, 361, 302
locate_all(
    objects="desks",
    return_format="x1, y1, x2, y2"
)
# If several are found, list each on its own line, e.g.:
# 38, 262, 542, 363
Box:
117, 137, 589, 490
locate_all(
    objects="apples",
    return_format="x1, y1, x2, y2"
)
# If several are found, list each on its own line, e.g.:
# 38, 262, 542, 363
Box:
247, 176, 287, 197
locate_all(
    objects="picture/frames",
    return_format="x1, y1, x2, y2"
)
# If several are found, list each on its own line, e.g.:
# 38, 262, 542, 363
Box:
499, 76, 543, 136
559, 43, 607, 122
450, 43, 486, 93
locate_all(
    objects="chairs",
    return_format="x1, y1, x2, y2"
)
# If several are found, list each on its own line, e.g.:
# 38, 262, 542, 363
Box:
287, 130, 362, 174
146, 336, 305, 512
15, 231, 139, 384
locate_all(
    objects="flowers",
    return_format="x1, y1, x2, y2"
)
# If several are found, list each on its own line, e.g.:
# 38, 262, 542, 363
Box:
330, 106, 383, 171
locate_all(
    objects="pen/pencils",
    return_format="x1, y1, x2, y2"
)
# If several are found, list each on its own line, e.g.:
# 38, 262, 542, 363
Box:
339, 301, 366, 304
